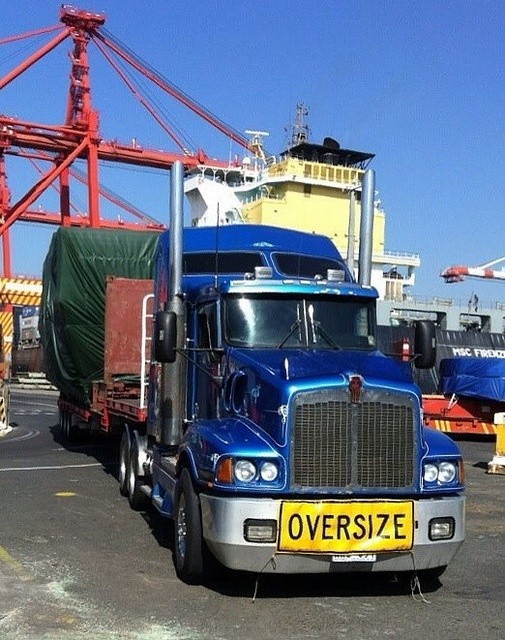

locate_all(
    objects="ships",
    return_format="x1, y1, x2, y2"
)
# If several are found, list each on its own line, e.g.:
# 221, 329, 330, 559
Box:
183, 103, 505, 398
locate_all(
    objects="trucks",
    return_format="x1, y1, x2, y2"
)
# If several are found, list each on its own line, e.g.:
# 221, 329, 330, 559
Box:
55, 159, 467, 590
